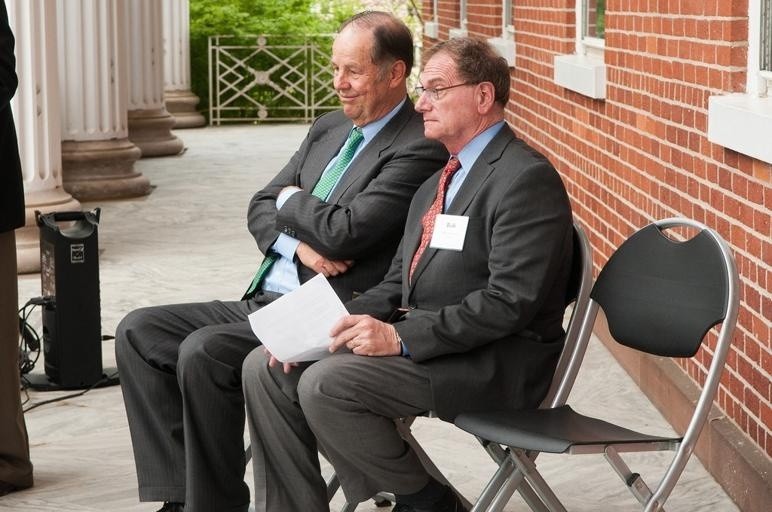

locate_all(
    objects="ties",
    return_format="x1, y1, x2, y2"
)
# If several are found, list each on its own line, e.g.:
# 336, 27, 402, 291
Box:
409, 157, 461, 288
247, 128, 363, 296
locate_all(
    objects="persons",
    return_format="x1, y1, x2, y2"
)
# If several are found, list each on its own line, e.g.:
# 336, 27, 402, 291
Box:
240, 34, 576, 512
114, 10, 450, 512
0, 0, 35, 500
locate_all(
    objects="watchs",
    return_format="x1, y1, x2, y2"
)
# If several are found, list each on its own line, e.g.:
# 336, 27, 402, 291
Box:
396, 332, 403, 356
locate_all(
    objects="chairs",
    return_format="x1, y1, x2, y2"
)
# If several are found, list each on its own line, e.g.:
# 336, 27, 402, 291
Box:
340, 217, 595, 511
246, 440, 340, 512
452, 217, 740, 511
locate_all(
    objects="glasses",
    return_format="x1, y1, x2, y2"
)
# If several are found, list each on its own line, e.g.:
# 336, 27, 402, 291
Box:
415, 83, 463, 100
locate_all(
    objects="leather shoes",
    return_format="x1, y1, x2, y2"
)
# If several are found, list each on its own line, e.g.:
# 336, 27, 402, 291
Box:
391, 485, 464, 512
